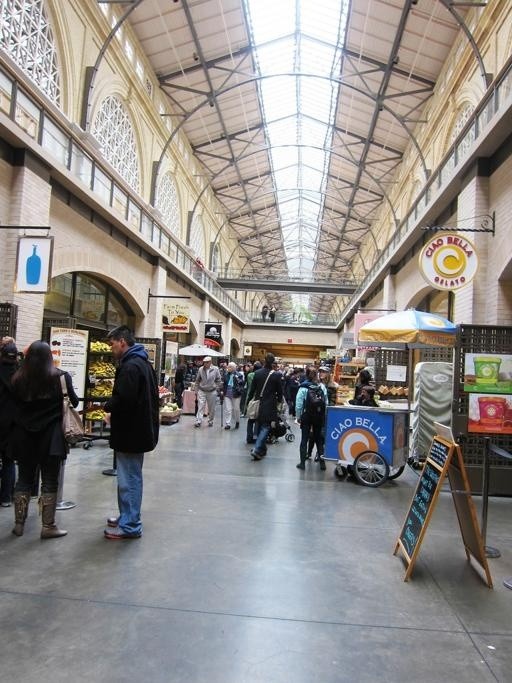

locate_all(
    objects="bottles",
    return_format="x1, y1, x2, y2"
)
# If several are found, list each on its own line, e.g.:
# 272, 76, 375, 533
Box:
472, 356, 503, 385
478, 396, 507, 425
26, 242, 41, 285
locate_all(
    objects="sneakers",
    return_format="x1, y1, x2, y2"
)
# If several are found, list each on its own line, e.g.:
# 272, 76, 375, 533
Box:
107, 515, 142, 527
296, 461, 306, 470
250, 447, 267, 460
101, 525, 145, 540
224, 425, 231, 430
234, 421, 239, 429
208, 421, 213, 426
194, 422, 200, 427
319, 460, 327, 471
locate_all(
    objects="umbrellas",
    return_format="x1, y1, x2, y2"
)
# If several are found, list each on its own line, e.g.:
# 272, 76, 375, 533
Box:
179, 344, 226, 357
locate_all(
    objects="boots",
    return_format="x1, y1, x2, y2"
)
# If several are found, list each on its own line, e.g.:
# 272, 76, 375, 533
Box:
11, 483, 31, 536
36, 490, 68, 539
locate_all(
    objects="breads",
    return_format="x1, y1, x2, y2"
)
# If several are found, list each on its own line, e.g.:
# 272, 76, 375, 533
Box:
378, 385, 409, 396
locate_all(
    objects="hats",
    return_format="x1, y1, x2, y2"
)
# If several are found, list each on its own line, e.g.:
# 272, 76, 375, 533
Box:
202, 357, 212, 362
317, 365, 332, 372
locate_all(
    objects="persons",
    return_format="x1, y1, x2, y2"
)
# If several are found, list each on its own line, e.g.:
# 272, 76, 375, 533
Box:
0, 336, 77, 538
173, 353, 338, 470
351, 386, 380, 407
359, 307, 458, 409
354, 370, 372, 386
262, 303, 268, 322
103, 325, 159, 539
269, 305, 276, 322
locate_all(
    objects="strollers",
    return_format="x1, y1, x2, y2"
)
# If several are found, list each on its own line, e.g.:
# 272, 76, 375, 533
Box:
266, 402, 296, 445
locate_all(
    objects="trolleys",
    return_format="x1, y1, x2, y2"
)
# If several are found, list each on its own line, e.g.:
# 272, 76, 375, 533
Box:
318, 400, 415, 488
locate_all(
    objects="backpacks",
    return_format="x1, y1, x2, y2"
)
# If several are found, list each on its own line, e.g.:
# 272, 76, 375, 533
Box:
298, 383, 326, 420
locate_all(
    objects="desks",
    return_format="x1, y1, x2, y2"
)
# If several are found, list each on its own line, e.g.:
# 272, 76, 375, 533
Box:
182, 388, 210, 416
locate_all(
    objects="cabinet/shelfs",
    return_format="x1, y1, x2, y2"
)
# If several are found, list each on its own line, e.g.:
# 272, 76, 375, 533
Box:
337, 361, 367, 390
82, 337, 119, 441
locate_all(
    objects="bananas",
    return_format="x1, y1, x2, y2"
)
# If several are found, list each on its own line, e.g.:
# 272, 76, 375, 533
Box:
86, 341, 116, 419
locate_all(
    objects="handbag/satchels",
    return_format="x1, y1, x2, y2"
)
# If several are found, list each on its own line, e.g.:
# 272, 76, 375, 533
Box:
247, 396, 261, 421
58, 372, 86, 444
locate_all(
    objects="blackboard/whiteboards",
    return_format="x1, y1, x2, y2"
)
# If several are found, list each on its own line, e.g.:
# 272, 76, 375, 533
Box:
398, 436, 454, 561
